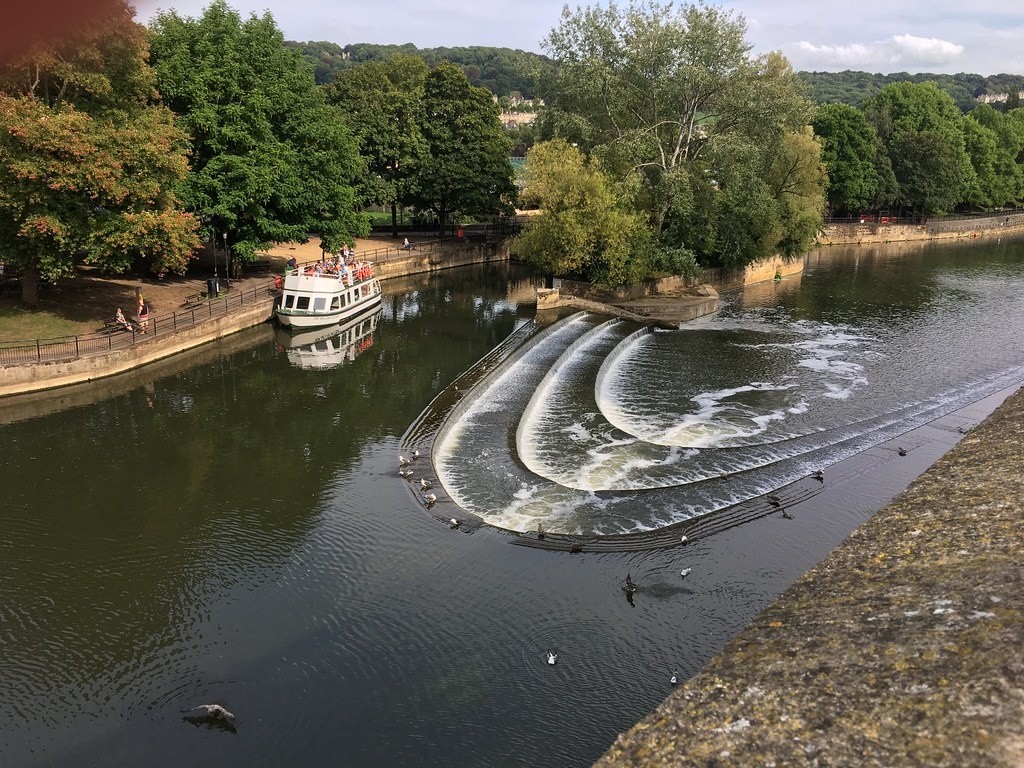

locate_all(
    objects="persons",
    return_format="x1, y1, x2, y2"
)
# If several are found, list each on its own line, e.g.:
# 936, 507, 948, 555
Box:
115, 286, 149, 335
1006, 217, 1009, 225
274, 246, 370, 295
846, 212, 852, 224
403, 217, 464, 251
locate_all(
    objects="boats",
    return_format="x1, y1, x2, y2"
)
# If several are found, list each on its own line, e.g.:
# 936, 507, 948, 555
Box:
277, 298, 384, 371
274, 257, 382, 327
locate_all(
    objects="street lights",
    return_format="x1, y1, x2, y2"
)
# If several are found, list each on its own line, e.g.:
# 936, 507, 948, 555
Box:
222, 228, 230, 292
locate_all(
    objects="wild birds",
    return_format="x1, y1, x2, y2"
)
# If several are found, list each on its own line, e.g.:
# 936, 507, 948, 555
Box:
537, 522, 547, 539
621, 574, 636, 592
194, 703, 235, 722
681, 535, 687, 542
397, 450, 460, 528
568, 541, 583, 551
767, 493, 795, 520
680, 567, 691, 576
670, 668, 679, 684
812, 469, 824, 476
546, 651, 557, 664
898, 445, 908, 457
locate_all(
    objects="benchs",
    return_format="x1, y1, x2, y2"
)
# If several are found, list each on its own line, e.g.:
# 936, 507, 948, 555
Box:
184, 295, 200, 303
104, 315, 136, 333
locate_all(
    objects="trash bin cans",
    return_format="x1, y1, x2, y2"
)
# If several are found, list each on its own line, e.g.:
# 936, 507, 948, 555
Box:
207, 278, 220, 298
